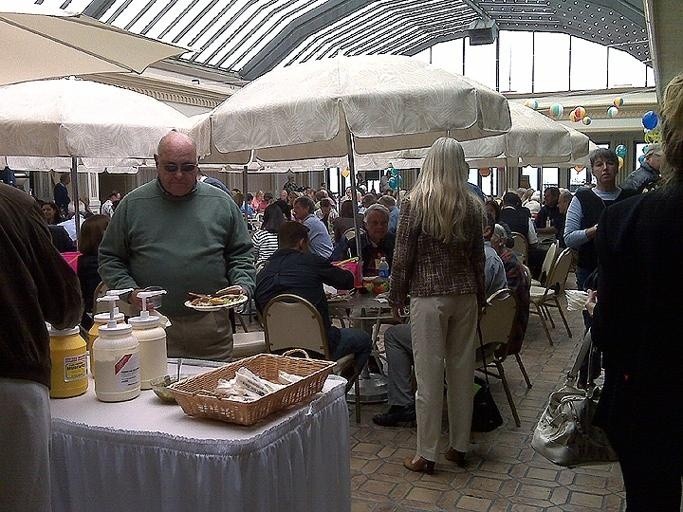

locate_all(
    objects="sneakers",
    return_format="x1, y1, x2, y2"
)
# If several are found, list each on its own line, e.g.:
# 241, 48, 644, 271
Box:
372, 411, 415, 428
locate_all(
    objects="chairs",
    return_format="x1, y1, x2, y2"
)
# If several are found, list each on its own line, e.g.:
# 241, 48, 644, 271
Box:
496, 262, 534, 389
530, 247, 572, 346
530, 240, 560, 286
263, 294, 361, 423
344, 227, 366, 259
474, 288, 521, 427
80, 280, 132, 342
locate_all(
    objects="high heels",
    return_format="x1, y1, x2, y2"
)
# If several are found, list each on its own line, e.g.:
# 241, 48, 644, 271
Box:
403, 455, 436, 475
444, 447, 470, 468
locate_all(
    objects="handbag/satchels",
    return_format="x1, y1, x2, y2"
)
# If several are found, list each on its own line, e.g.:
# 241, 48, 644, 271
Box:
530, 384, 597, 467
329, 257, 363, 289
443, 368, 503, 432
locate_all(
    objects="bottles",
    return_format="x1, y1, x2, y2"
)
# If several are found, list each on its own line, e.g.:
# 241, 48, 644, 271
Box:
378, 257, 390, 292
545, 217, 550, 228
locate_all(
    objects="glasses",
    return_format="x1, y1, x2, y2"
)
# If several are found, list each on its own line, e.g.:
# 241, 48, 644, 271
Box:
156, 163, 198, 172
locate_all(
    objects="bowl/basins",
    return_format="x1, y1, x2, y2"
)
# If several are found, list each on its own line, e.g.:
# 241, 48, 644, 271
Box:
149, 375, 202, 406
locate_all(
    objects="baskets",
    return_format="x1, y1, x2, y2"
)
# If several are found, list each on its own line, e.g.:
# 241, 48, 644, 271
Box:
165, 348, 337, 423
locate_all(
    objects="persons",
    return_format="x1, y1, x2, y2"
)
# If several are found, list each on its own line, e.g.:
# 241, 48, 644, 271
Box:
0, 72, 683, 511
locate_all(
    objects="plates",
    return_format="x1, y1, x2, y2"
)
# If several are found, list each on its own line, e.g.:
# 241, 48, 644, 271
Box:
184, 295, 249, 313
